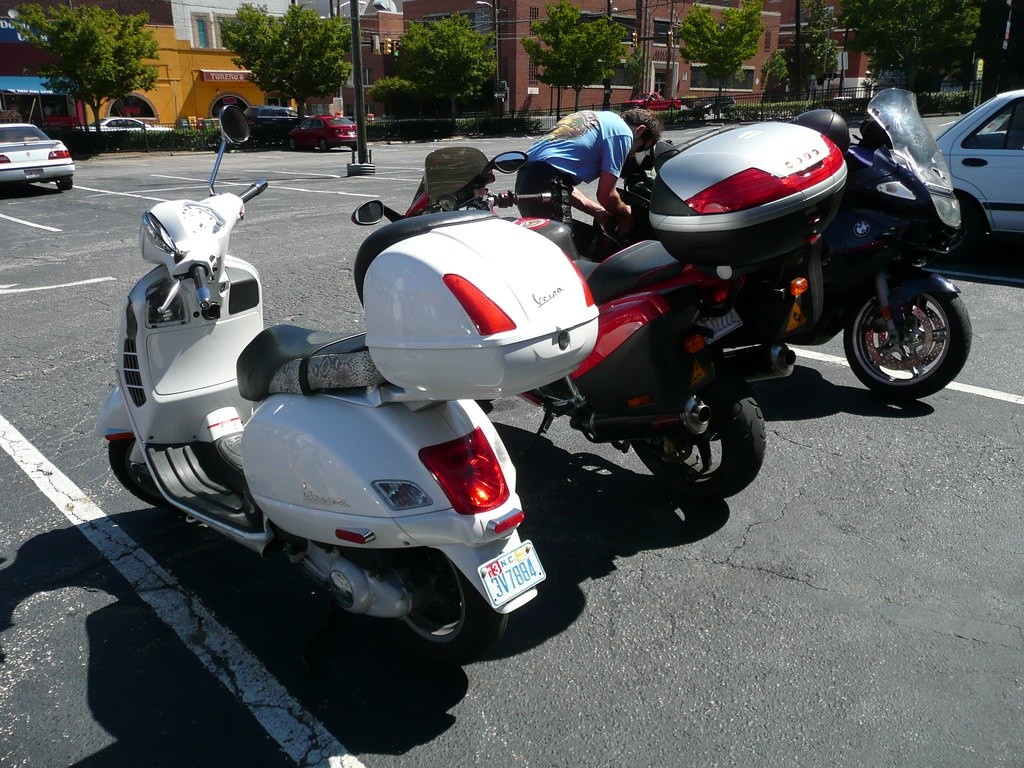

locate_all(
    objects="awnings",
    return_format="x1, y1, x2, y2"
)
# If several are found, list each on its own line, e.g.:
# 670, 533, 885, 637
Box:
0, 75, 79, 96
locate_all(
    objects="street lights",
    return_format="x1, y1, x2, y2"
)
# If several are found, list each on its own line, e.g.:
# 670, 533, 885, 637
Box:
476, 0, 500, 117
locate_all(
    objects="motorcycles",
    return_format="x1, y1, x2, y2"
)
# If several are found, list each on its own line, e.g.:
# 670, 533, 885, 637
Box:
573, 87, 973, 401
349, 119, 850, 506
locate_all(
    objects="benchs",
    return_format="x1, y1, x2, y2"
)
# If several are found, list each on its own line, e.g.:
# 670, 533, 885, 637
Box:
966, 129, 1024, 149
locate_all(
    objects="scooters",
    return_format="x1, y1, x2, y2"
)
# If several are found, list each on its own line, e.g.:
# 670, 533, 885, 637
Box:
94, 102, 601, 672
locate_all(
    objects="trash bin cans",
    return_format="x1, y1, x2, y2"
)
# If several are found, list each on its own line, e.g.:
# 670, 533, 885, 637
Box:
499, 81, 509, 114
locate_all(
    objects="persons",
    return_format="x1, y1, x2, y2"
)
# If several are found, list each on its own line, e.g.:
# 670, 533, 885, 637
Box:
514, 109, 661, 236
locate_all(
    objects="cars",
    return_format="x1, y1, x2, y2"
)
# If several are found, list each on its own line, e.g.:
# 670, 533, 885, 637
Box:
928, 89, 1024, 233
287, 113, 357, 152
74, 116, 175, 132
693, 96, 737, 120
0, 122, 76, 190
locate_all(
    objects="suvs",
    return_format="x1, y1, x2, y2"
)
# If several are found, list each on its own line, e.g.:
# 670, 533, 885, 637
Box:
242, 103, 315, 127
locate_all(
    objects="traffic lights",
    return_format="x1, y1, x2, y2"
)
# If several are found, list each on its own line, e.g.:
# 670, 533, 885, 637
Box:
666, 31, 673, 47
393, 40, 401, 57
630, 32, 637, 47
383, 38, 392, 55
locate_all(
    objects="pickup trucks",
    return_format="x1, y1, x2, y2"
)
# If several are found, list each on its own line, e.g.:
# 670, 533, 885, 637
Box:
620, 90, 682, 111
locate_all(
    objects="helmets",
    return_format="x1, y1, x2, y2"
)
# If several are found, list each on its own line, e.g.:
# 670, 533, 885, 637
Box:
786, 109, 851, 156
